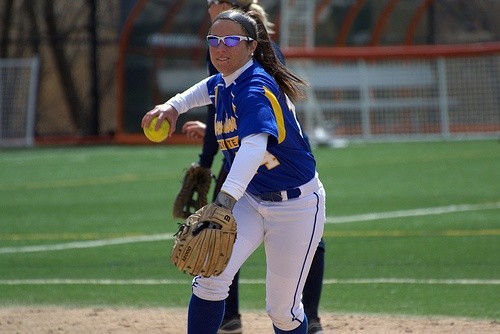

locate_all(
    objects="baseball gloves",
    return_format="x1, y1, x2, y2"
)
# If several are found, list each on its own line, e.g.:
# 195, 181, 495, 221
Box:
172, 162, 215, 221
168, 191, 238, 279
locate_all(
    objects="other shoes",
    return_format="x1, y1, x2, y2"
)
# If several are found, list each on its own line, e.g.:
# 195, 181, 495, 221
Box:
219, 314, 242, 334
308, 316, 324, 334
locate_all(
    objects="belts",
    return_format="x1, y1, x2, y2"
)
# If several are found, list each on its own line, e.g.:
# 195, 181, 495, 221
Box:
258, 188, 301, 202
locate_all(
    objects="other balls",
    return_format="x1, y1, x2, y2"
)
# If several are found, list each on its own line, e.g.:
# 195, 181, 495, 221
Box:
142, 115, 171, 143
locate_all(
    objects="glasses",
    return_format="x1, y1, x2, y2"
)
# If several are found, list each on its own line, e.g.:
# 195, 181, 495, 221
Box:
207, 35, 255, 47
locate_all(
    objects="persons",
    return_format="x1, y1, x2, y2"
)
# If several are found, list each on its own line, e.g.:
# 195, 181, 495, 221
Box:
141, 9, 326, 334
198, 0, 326, 334
